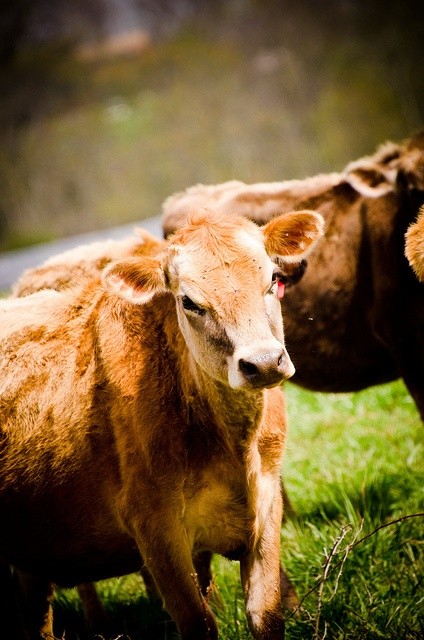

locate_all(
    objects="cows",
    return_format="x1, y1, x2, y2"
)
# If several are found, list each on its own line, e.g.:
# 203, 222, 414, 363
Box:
0, 206, 325, 640
11, 228, 307, 634
161, 131, 423, 426
